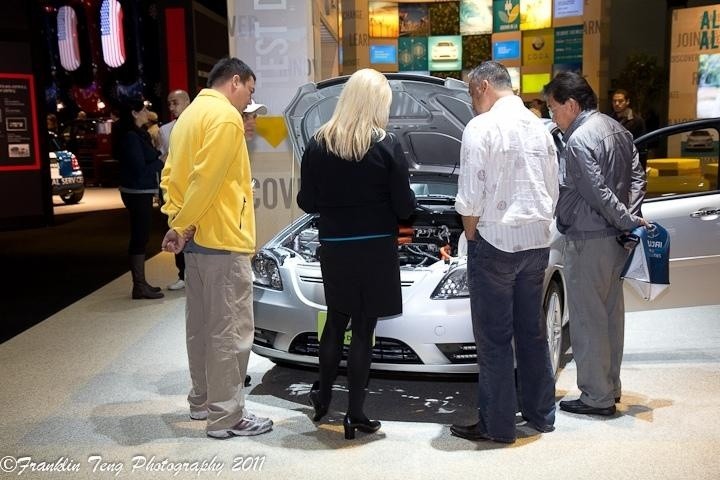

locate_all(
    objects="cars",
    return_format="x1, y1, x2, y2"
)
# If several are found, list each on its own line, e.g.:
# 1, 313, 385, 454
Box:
430, 40, 460, 63
685, 129, 714, 152
47, 130, 87, 206
250, 61, 720, 393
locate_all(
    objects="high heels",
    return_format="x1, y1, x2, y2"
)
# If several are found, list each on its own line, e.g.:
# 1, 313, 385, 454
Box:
310, 381, 331, 421
343, 412, 381, 440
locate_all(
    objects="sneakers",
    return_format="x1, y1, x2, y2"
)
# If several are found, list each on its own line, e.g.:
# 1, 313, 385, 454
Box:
190, 408, 209, 419
207, 411, 273, 437
168, 280, 186, 290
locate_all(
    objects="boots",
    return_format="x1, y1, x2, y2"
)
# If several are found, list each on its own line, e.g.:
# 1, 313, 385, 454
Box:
127, 254, 164, 299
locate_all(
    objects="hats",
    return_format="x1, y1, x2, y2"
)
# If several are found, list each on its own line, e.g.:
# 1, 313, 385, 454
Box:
242, 98, 268, 115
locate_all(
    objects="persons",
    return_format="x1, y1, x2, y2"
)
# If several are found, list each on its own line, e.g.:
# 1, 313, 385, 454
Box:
295, 68, 417, 440
159, 56, 274, 440
114, 96, 168, 300
449, 59, 561, 443
150, 89, 190, 290
241, 98, 268, 143
46, 110, 121, 153
543, 71, 652, 416
529, 97, 550, 119
609, 90, 647, 173
145, 112, 161, 204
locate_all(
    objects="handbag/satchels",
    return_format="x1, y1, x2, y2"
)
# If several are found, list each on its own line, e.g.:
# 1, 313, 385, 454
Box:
617, 222, 670, 302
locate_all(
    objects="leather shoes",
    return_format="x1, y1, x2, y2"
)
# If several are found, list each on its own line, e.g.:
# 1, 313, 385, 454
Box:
560, 399, 616, 416
450, 421, 515, 443
